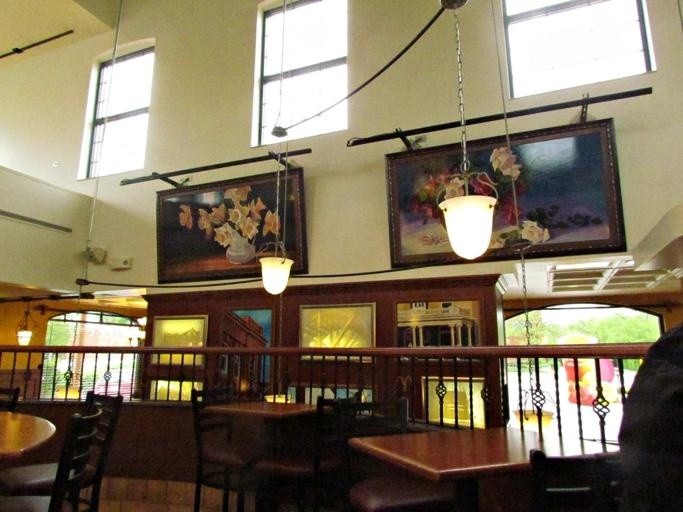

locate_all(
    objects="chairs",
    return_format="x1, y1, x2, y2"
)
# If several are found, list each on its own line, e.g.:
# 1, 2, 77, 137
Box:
0, 392, 123, 512
341, 399, 459, 511
529, 450, 622, 512
193, 388, 265, 512
255, 389, 361, 512
0, 403, 103, 511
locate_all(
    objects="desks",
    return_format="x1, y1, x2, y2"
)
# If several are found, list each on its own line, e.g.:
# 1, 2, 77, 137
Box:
0, 412, 56, 459
206, 402, 332, 512
353, 428, 620, 512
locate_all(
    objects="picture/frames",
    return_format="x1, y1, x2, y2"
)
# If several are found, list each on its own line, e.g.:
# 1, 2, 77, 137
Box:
156, 169, 307, 284
385, 119, 628, 270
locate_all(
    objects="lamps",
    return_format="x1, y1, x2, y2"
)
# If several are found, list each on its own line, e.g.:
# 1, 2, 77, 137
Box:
260, 126, 293, 295
16, 298, 32, 347
435, 0, 499, 259
